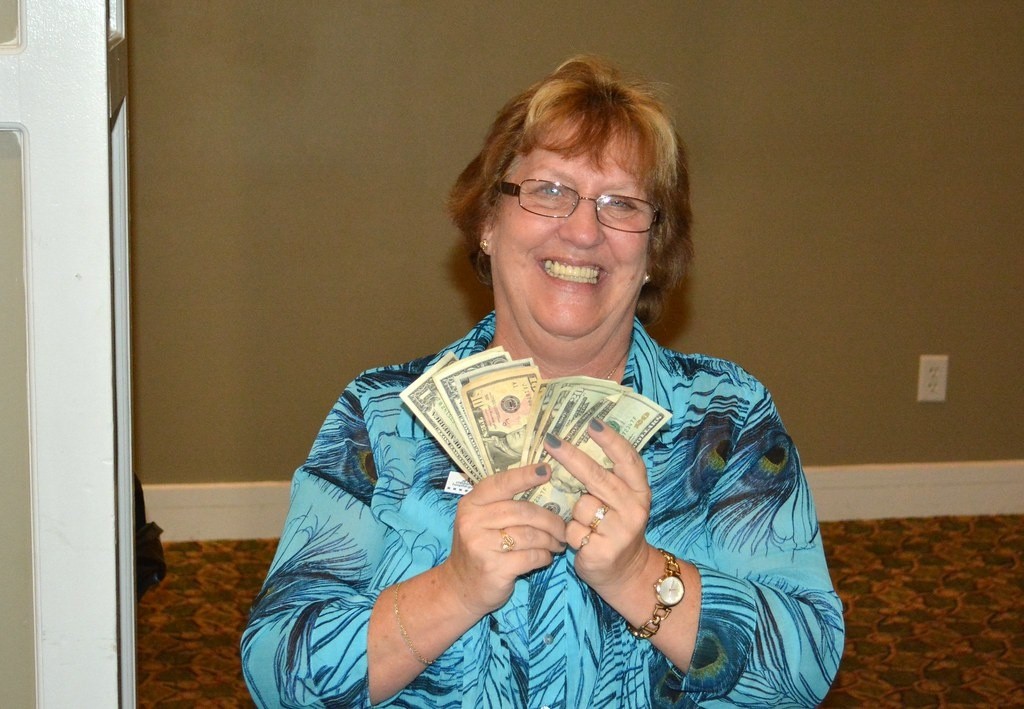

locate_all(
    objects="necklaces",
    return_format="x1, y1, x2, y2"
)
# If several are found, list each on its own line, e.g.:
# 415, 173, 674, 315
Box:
607, 366, 617, 379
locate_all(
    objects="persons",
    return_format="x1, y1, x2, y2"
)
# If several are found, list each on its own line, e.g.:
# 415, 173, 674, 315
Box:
238, 57, 845, 709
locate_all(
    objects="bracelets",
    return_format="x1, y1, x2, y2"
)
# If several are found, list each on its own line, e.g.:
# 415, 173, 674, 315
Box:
394, 580, 437, 665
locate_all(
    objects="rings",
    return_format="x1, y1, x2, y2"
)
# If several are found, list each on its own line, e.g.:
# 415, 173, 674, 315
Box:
500, 529, 515, 551
577, 529, 593, 550
589, 505, 609, 530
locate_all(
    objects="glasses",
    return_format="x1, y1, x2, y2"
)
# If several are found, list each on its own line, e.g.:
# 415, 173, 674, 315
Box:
498, 178, 660, 234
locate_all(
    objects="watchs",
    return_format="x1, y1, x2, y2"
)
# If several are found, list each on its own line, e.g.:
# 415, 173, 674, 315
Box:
626, 548, 685, 639
